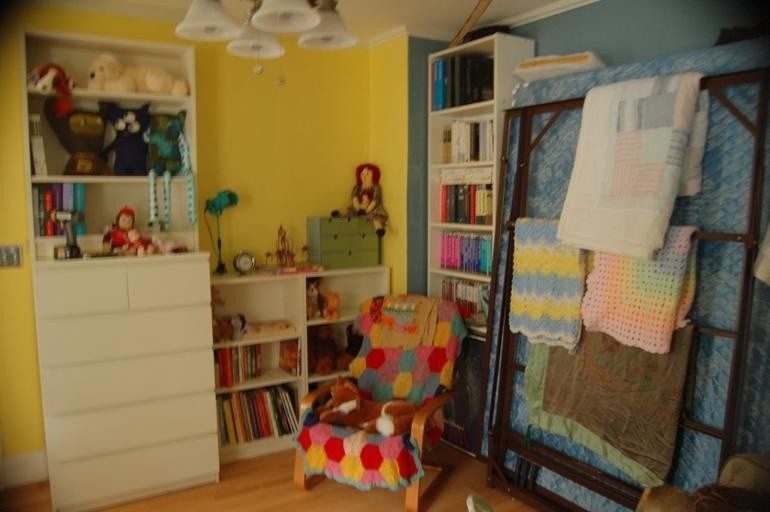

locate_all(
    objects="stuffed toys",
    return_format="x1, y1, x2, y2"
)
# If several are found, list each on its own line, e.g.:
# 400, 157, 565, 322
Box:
306, 278, 340, 319
88, 54, 190, 98
319, 375, 420, 437
42, 95, 191, 179
309, 325, 353, 375
28, 65, 75, 116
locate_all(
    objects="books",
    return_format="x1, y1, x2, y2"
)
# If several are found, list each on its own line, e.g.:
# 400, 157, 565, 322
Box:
215, 340, 301, 445
432, 52, 493, 325
33, 184, 86, 237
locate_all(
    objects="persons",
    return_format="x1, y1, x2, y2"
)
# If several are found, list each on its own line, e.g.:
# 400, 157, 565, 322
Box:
109, 208, 154, 256
329, 164, 387, 236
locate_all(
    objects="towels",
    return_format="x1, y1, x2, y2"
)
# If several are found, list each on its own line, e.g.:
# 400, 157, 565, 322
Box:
518, 322, 698, 490
507, 215, 590, 355
554, 72, 713, 259
581, 224, 703, 355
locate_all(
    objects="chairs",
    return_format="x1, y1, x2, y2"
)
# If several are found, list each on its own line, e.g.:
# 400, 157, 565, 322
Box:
293, 291, 468, 512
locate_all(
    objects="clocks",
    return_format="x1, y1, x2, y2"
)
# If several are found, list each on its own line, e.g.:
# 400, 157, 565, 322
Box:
235, 250, 255, 275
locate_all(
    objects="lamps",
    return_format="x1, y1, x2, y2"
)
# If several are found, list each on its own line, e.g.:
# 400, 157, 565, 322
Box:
173, 0, 357, 58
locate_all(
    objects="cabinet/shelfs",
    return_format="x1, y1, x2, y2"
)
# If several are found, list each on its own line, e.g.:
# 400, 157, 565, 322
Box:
23, 32, 222, 511
425, 33, 537, 459
483, 65, 770, 512
211, 266, 390, 464
306, 214, 380, 269
